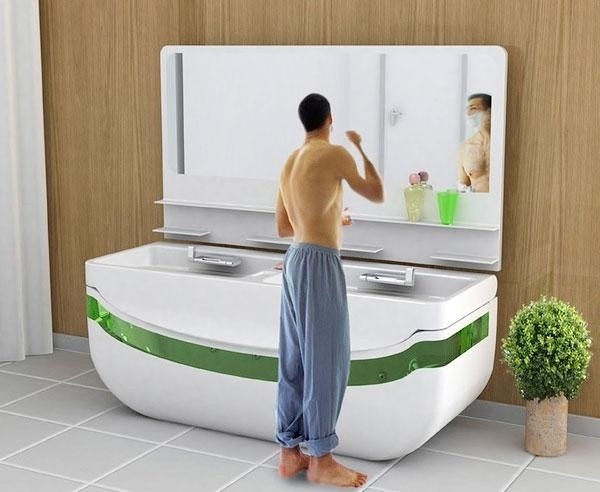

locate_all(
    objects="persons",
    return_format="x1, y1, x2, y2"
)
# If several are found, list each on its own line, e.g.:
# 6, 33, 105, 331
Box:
273, 90, 385, 489
457, 92, 491, 192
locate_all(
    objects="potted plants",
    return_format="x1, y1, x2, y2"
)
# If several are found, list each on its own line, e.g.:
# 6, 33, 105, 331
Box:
499, 293, 595, 457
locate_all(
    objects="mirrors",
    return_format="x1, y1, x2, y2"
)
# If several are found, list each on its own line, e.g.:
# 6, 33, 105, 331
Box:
159, 45, 510, 274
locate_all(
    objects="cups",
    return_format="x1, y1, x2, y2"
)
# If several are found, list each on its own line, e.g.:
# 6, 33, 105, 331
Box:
436, 191, 458, 225
341, 206, 353, 226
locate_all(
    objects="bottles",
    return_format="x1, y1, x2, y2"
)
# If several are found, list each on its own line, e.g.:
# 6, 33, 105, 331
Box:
418, 171, 433, 191
404, 172, 424, 222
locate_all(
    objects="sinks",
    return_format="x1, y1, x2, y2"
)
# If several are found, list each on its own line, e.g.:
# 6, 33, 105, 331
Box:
343, 264, 475, 306
95, 244, 282, 282
